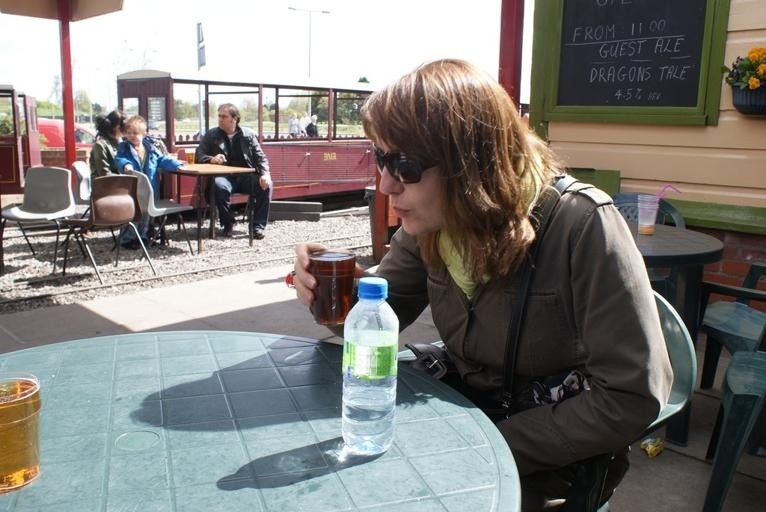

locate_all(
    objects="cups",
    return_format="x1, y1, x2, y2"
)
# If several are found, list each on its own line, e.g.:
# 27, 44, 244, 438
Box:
186, 153, 195, 165
0, 371, 46, 493
309, 248, 358, 326
638, 195, 660, 236
169, 153, 177, 160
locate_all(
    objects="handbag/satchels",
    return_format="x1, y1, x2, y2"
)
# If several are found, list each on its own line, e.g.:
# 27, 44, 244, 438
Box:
501, 366, 630, 507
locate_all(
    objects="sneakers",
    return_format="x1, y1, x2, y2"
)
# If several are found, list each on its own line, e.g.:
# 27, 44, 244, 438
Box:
253, 225, 265, 239
222, 216, 234, 238
120, 229, 160, 250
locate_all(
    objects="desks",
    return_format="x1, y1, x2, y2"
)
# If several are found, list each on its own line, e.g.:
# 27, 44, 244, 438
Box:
160, 164, 256, 254
0, 330, 522, 512
627, 222, 724, 351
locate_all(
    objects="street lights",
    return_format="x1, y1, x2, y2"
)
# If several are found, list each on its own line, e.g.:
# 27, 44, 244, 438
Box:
289, 5, 331, 118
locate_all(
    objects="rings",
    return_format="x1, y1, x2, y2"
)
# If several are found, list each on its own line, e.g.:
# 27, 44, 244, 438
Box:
286, 270, 296, 288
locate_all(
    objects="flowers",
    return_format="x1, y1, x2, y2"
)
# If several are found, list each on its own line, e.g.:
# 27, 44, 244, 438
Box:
720, 44, 766, 90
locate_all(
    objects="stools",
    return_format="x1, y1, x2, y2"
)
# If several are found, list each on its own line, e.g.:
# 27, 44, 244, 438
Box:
397, 288, 698, 512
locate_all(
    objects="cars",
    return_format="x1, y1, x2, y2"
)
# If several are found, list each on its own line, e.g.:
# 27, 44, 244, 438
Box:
36, 117, 98, 148
148, 120, 158, 130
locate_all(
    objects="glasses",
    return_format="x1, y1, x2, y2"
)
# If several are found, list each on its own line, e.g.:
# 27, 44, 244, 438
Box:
372, 142, 442, 184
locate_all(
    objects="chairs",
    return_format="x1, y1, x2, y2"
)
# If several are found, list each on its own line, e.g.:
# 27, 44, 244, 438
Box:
57, 174, 157, 284
201, 193, 256, 241
702, 351, 766, 512
609, 192, 685, 306
60, 160, 120, 246
0, 167, 86, 276
111, 169, 194, 260
699, 262, 766, 389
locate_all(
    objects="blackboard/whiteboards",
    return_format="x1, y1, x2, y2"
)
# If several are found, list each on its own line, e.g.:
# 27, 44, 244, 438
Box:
543, 0, 731, 125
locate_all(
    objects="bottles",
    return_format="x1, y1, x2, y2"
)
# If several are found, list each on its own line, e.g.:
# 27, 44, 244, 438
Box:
340, 277, 398, 454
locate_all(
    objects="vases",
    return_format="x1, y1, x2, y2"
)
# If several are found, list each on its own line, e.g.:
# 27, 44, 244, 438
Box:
732, 86, 766, 113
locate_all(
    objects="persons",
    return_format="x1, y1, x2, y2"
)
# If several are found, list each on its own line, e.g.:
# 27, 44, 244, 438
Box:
293, 60, 674, 512
193, 103, 273, 239
90, 110, 128, 188
116, 116, 185, 250
289, 111, 319, 138
